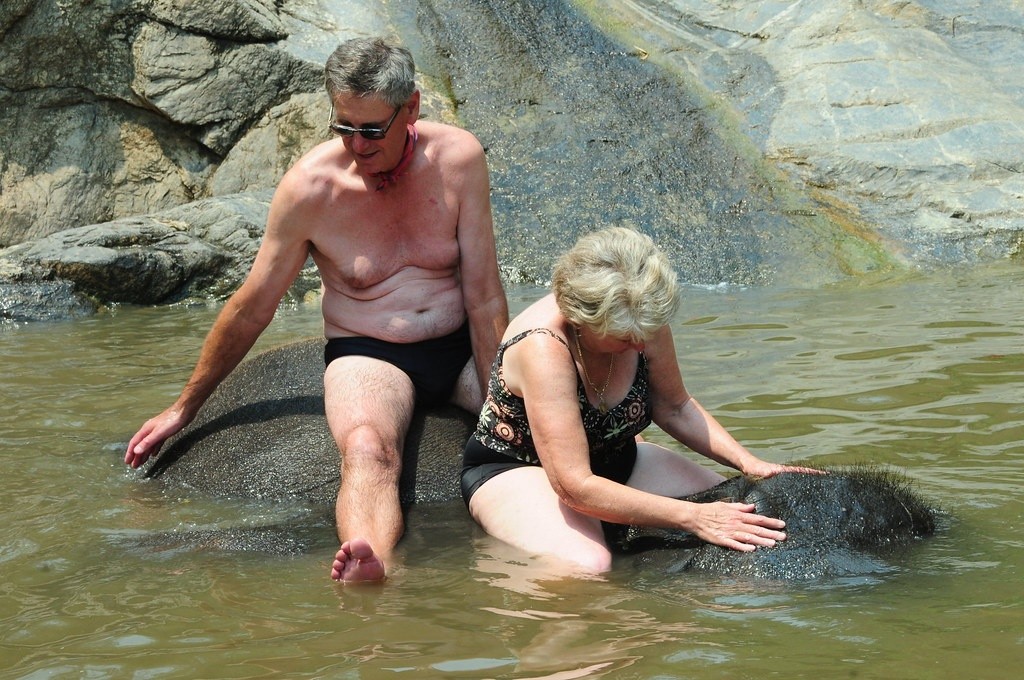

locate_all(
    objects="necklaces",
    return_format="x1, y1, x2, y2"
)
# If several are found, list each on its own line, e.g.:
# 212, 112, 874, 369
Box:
572, 327, 616, 413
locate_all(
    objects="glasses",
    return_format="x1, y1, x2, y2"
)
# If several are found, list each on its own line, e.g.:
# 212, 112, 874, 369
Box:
327, 104, 403, 140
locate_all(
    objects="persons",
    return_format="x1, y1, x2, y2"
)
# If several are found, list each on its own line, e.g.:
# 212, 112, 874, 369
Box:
460, 226, 829, 577
124, 34, 646, 582
